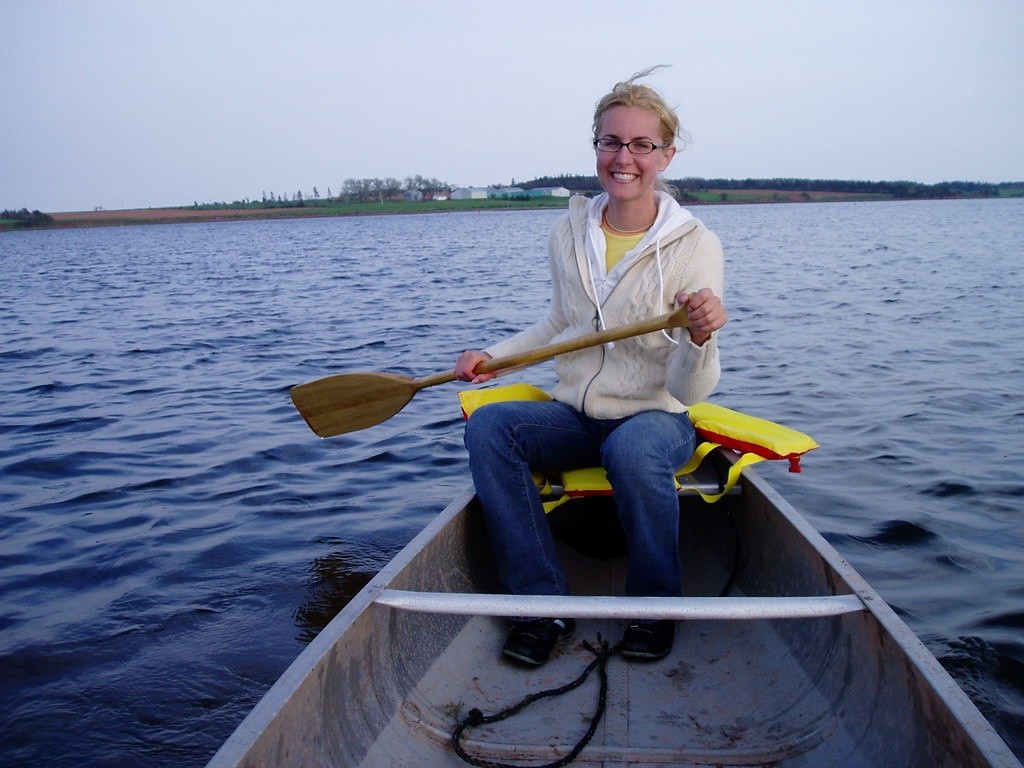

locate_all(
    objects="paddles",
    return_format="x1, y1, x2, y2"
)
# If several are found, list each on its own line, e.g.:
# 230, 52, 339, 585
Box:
289, 300, 694, 439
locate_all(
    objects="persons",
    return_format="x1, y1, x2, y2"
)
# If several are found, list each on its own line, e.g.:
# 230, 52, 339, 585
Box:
455, 84, 727, 667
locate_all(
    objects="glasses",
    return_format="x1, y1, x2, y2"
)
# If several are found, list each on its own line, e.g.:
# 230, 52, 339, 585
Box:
594, 139, 669, 154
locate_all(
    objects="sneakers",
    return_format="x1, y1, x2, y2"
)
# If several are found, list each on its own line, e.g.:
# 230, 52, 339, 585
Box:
502, 617, 577, 669
619, 620, 677, 661
